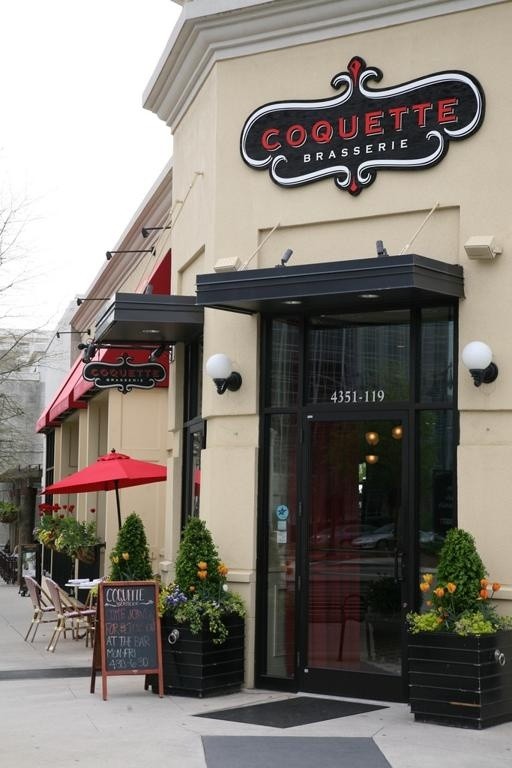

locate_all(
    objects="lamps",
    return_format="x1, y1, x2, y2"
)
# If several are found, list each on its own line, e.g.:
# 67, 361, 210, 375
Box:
206, 354, 242, 395
461, 341, 497, 387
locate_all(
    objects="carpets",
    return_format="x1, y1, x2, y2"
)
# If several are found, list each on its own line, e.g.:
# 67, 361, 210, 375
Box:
191, 696, 390, 729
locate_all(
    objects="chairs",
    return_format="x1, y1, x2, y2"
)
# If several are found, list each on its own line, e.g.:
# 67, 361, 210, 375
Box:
24, 576, 82, 642
45, 577, 95, 652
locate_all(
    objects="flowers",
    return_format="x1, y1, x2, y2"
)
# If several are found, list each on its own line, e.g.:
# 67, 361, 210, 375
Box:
157, 559, 246, 644
33, 501, 100, 557
406, 573, 512, 635
112, 551, 135, 580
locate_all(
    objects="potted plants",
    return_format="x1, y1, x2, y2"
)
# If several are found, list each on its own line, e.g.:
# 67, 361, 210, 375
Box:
152, 517, 247, 699
404, 527, 512, 730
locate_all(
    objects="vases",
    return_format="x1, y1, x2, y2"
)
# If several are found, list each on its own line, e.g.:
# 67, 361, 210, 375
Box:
36, 543, 106, 604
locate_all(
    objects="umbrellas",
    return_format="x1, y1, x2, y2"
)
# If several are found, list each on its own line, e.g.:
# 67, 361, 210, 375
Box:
39, 447, 167, 538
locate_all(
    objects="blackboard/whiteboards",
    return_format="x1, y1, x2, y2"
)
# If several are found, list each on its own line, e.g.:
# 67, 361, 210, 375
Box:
100, 581, 162, 676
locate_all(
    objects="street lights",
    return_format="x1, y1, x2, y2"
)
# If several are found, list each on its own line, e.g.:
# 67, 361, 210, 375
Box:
360, 432, 381, 523
392, 426, 403, 441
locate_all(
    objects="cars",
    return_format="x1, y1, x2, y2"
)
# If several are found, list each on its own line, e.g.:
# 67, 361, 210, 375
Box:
280, 523, 446, 623
